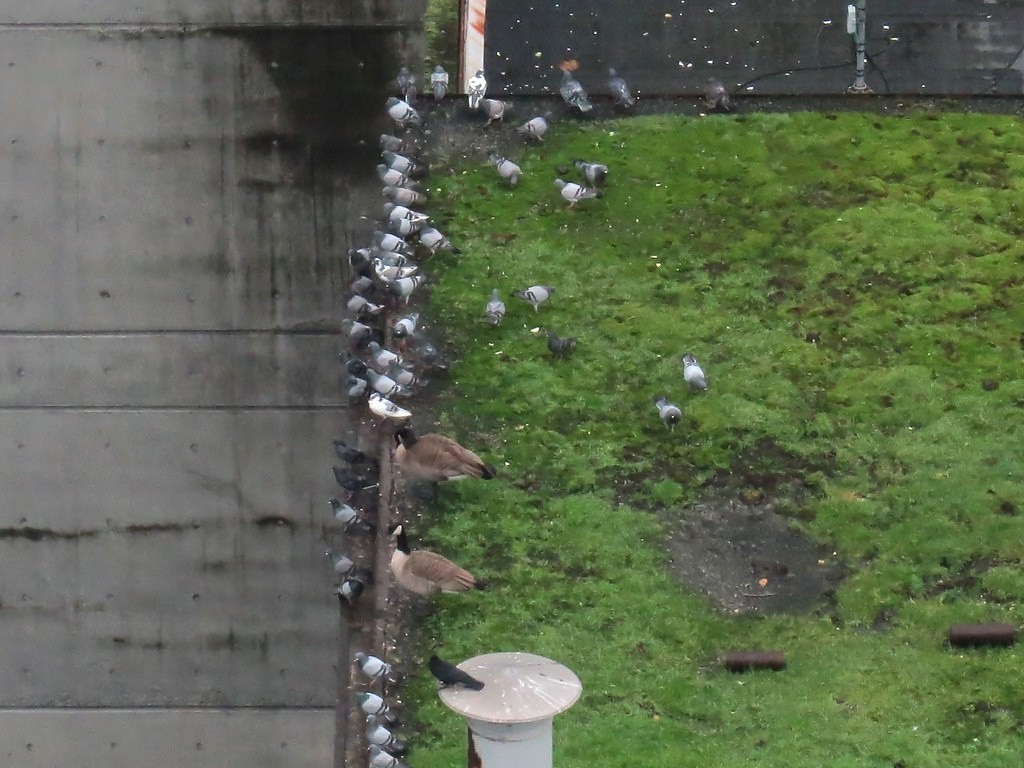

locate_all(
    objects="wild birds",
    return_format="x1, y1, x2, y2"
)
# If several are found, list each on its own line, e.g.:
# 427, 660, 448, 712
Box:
683, 353, 708, 394
608, 68, 637, 109
432, 63, 449, 102
548, 331, 578, 362
487, 150, 523, 186
510, 285, 558, 315
467, 69, 488, 109
325, 93, 461, 768
395, 428, 496, 498
560, 70, 596, 113
516, 116, 548, 143
577, 158, 609, 188
554, 178, 610, 208
655, 396, 682, 433
427, 653, 486, 691
484, 288, 506, 327
388, 521, 489, 613
477, 98, 515, 127
703, 76, 739, 112
